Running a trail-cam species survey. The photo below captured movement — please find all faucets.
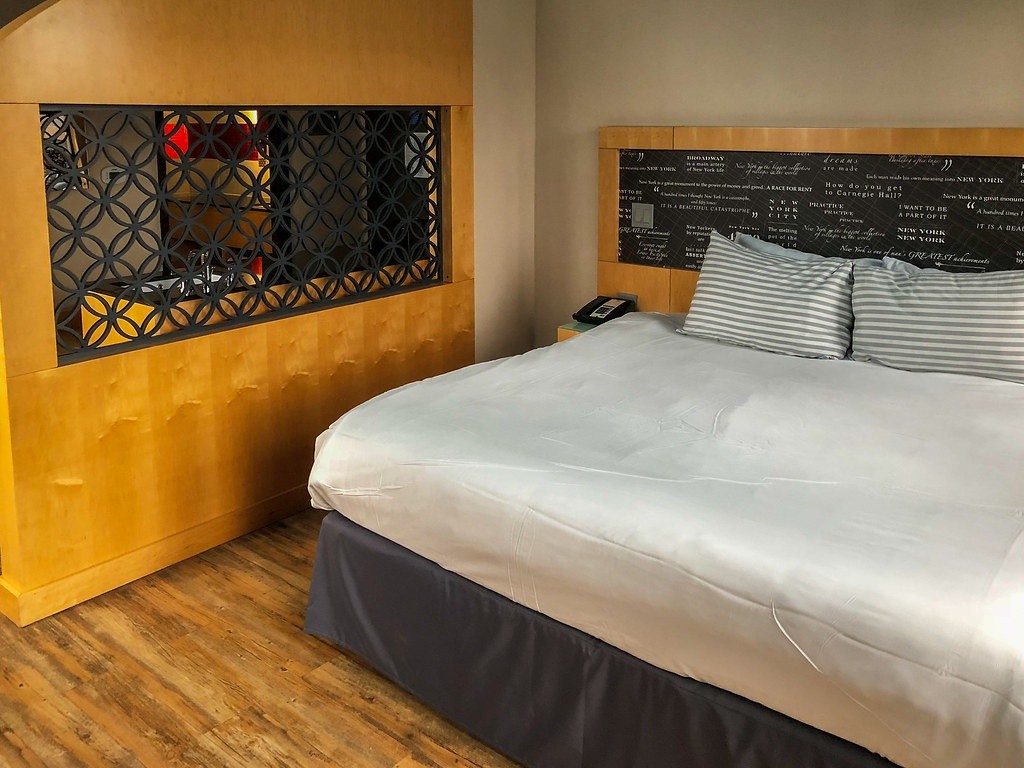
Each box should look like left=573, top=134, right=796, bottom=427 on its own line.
left=189, top=250, right=211, bottom=293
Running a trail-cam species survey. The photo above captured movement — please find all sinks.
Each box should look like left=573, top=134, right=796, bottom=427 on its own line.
left=110, top=269, right=244, bottom=292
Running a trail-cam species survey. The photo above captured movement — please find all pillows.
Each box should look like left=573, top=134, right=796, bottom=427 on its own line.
left=675, top=229, right=1024, bottom=385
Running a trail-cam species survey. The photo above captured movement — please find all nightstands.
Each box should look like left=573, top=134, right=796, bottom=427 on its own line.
left=557, top=321, right=597, bottom=342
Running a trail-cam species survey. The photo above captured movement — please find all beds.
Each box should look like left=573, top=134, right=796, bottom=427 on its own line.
left=304, top=310, right=1024, bottom=768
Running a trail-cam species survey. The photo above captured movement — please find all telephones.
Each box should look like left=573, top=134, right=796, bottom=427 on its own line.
left=572, top=295, right=633, bottom=326
